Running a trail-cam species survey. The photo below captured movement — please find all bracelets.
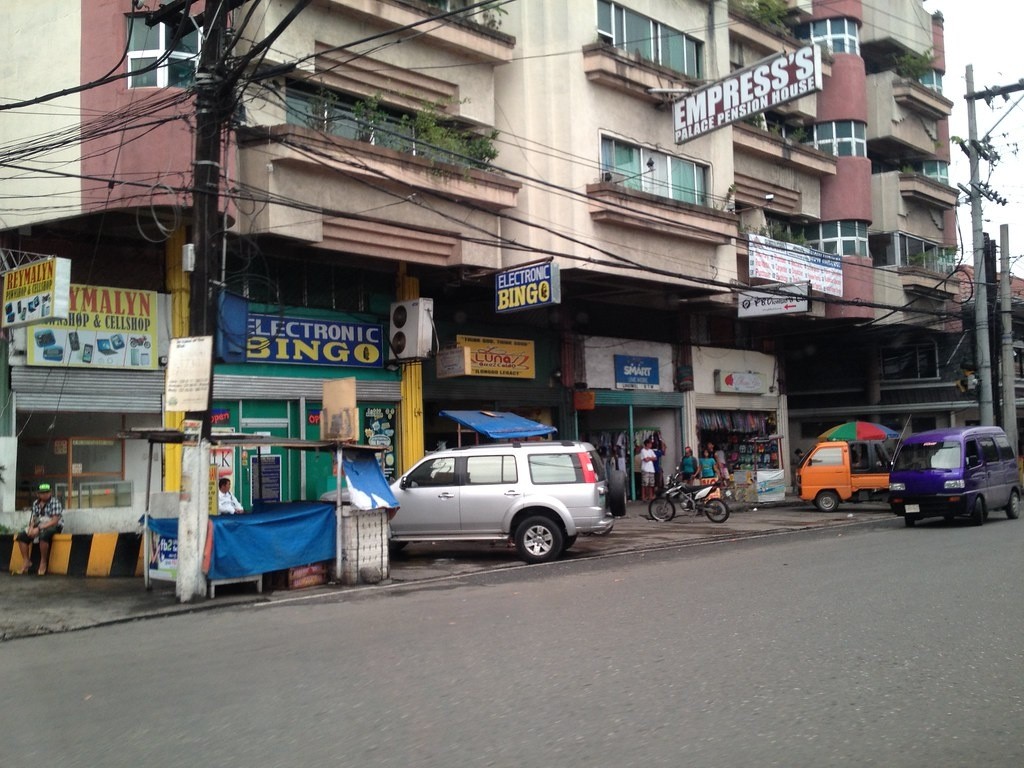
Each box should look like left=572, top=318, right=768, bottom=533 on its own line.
left=38, top=526, right=41, bottom=531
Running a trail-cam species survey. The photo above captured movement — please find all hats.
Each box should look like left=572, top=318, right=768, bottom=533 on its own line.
left=38, top=484, right=51, bottom=493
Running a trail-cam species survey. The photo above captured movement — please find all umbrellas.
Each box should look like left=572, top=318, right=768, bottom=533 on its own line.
left=817, top=420, right=900, bottom=442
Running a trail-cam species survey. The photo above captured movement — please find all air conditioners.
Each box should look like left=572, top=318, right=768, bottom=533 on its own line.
left=388, top=298, right=433, bottom=361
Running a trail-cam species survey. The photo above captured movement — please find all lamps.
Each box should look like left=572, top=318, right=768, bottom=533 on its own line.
left=735, top=193, right=774, bottom=213
left=613, top=157, right=654, bottom=183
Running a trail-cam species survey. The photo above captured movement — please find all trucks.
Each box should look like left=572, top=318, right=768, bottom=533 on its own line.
left=794, top=439, right=891, bottom=514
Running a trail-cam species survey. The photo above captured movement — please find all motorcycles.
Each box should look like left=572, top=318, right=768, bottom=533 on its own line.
left=648, top=465, right=730, bottom=524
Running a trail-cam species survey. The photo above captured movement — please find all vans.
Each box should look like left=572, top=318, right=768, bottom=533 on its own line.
left=886, top=424, right=1022, bottom=527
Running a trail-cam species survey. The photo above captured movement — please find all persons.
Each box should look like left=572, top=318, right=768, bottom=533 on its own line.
left=16, top=483, right=64, bottom=575
left=679, top=447, right=697, bottom=485
left=690, top=449, right=720, bottom=479
left=217, top=478, right=245, bottom=515
left=794, top=448, right=804, bottom=461
left=639, top=439, right=657, bottom=500
left=705, top=442, right=728, bottom=486
left=651, top=443, right=665, bottom=491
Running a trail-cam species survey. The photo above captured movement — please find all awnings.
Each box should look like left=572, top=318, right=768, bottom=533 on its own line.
left=439, top=409, right=557, bottom=439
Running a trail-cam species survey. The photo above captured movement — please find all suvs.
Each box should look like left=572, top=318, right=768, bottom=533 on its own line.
left=319, top=437, right=628, bottom=564
